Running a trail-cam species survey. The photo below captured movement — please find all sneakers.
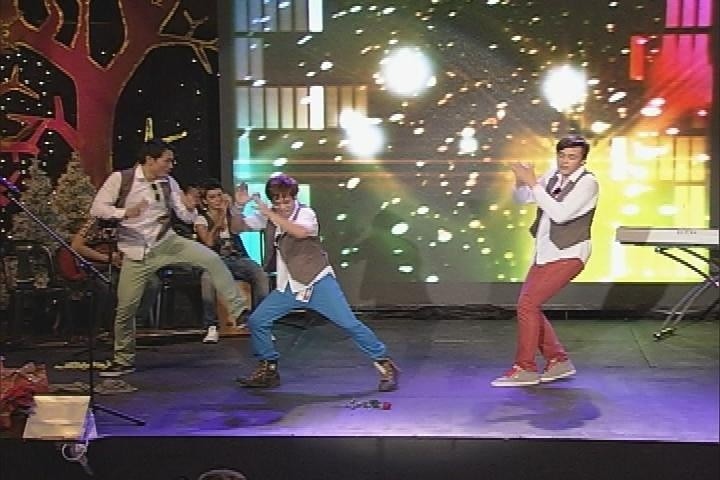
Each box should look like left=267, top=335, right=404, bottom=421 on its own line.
left=490, top=362, right=541, bottom=388
left=201, top=324, right=221, bottom=344
left=538, top=358, right=578, bottom=384
left=235, top=308, right=255, bottom=328
left=98, top=360, right=138, bottom=378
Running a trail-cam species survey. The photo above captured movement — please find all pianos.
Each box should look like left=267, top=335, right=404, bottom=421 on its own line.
left=615, top=226, right=720, bottom=245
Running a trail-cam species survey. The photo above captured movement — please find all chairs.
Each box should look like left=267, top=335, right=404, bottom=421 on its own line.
left=12, top=241, right=253, bottom=335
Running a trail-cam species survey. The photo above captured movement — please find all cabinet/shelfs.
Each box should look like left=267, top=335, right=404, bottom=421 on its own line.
left=616, top=244, right=720, bottom=339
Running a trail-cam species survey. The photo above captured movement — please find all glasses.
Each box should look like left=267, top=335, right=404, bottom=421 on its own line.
left=149, top=183, right=161, bottom=201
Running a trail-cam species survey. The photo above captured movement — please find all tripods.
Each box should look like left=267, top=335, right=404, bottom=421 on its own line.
left=0, top=185, right=148, bottom=426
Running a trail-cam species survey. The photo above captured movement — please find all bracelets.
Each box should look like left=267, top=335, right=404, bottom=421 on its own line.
left=230, top=201, right=245, bottom=217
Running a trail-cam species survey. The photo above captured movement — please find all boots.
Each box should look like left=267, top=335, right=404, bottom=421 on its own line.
left=238, top=359, right=282, bottom=390
left=376, top=354, right=399, bottom=392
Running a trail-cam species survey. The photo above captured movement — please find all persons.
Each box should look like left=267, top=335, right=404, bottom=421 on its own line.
left=230, top=176, right=402, bottom=394
left=194, top=182, right=278, bottom=345
left=490, top=135, right=600, bottom=388
left=88, top=137, right=258, bottom=379
left=67, top=208, right=125, bottom=351
